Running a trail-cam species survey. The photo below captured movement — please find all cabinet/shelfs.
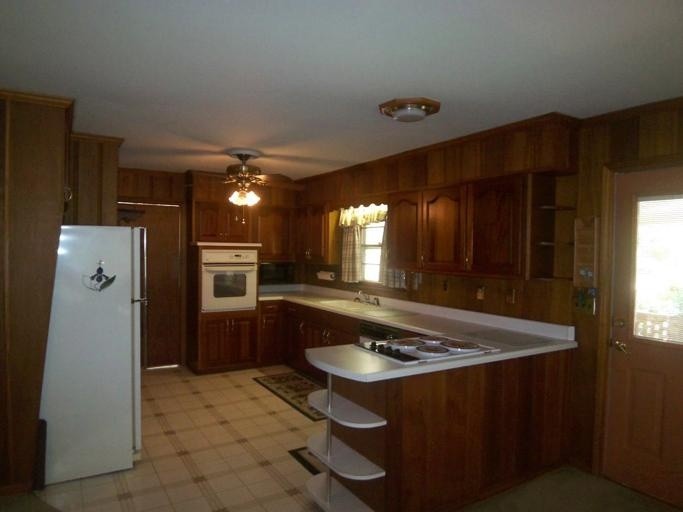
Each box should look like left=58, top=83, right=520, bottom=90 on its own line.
left=186, top=111, right=598, bottom=512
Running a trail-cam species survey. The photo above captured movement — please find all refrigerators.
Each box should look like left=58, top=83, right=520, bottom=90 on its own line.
left=37, top=224, right=150, bottom=489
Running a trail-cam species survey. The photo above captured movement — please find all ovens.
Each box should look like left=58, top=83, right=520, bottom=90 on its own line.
left=200, top=249, right=257, bottom=312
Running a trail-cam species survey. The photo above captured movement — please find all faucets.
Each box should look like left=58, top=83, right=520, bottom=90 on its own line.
left=362, top=292, right=370, bottom=303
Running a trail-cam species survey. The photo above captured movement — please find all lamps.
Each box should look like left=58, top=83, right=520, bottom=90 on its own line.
left=379, top=96, right=439, bottom=122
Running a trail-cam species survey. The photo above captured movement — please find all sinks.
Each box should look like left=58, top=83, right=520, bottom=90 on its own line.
left=319, top=299, right=380, bottom=311
